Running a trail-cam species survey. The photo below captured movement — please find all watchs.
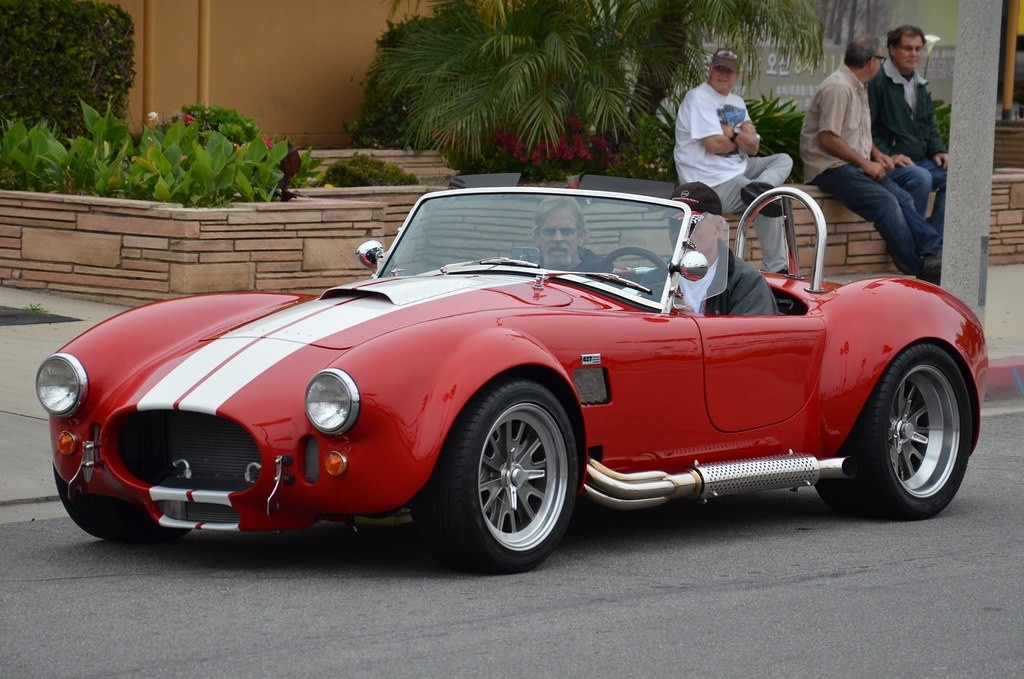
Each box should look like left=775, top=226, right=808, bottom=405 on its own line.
left=731, top=126, right=740, bottom=140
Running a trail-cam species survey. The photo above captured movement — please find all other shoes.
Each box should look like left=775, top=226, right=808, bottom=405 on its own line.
left=740, top=181, right=783, bottom=218
left=919, top=262, right=940, bottom=286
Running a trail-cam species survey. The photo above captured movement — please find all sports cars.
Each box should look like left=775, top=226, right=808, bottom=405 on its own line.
left=34, top=172, right=989, bottom=576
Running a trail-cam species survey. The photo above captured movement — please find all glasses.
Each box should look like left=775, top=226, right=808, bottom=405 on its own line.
left=538, top=225, right=581, bottom=236
left=867, top=54, right=887, bottom=65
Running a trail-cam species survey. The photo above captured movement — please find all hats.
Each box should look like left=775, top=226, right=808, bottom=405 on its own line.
left=660, top=181, right=722, bottom=220
left=713, top=48, right=738, bottom=72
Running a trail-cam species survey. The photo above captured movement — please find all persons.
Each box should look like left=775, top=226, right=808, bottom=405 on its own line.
left=528, top=196, right=615, bottom=274
left=673, top=48, right=793, bottom=274
left=639, top=181, right=779, bottom=317
left=867, top=25, right=949, bottom=235
left=799, top=37, right=943, bottom=286
